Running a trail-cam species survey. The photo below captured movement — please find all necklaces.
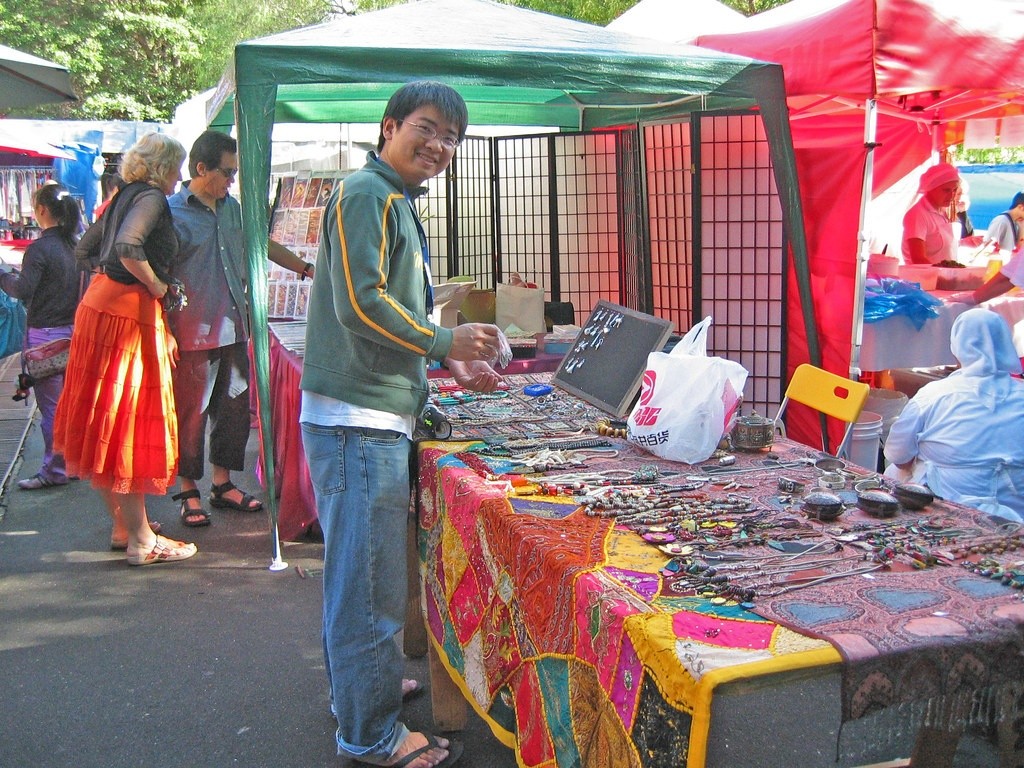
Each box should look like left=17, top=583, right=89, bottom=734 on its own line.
left=426, top=381, right=1024, bottom=611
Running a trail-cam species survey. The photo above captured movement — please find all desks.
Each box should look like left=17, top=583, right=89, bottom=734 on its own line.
left=859, top=296, right=1024, bottom=376
left=411, top=367, right=1024, bottom=768
left=249, top=322, right=575, bottom=542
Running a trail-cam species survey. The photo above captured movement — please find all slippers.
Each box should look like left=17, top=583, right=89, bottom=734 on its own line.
left=331, top=679, right=423, bottom=720
left=128, top=536, right=198, bottom=566
left=352, top=729, right=464, bottom=768
left=111, top=519, right=162, bottom=550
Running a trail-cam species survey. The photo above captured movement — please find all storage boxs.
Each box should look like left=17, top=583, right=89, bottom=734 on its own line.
left=543, top=332, right=577, bottom=355
left=510, top=337, right=537, bottom=358
left=553, top=323, right=581, bottom=334
left=505, top=330, right=538, bottom=338
left=908, top=264, right=990, bottom=291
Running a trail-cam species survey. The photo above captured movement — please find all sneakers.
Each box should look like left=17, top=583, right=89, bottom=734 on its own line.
left=19, top=471, right=54, bottom=489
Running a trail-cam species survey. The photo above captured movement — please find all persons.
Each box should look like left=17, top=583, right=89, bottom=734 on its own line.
left=988, top=192, right=1024, bottom=250
left=899, top=164, right=959, bottom=264
left=944, top=239, right=1024, bottom=305
left=54, top=133, right=197, bottom=566
left=883, top=308, right=1024, bottom=523
left=0, top=185, right=90, bottom=492
left=171, top=131, right=316, bottom=528
left=298, top=81, right=502, bottom=768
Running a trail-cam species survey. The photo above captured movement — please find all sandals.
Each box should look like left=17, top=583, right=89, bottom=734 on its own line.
left=172, top=488, right=210, bottom=527
left=209, top=479, right=262, bottom=511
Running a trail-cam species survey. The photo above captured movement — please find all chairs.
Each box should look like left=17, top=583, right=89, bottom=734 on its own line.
left=770, top=363, right=870, bottom=459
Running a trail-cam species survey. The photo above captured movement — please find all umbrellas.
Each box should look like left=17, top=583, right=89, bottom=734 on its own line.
left=1, top=46, right=78, bottom=116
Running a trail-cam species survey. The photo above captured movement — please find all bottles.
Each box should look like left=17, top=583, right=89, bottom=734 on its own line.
left=985, top=244, right=1003, bottom=282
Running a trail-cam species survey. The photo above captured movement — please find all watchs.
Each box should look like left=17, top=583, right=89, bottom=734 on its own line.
left=301, top=263, right=314, bottom=281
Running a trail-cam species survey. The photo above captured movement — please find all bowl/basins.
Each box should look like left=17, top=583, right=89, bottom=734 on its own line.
left=929, top=265, right=987, bottom=291
left=867, top=256, right=901, bottom=277
left=898, top=263, right=941, bottom=291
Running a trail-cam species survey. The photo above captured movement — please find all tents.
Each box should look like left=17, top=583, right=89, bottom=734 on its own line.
left=207, top=0, right=1024, bottom=570
left=0, top=119, right=171, bottom=271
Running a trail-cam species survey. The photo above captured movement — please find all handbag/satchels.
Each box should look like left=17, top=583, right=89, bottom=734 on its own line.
left=22, top=336, right=73, bottom=379
left=496, top=272, right=547, bottom=336
left=627, top=315, right=748, bottom=463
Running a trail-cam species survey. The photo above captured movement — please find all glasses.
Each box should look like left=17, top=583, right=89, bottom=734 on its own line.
left=398, top=119, right=459, bottom=150
left=217, top=165, right=238, bottom=178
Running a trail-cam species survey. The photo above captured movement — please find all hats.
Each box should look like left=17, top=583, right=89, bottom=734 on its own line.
left=918, top=162, right=959, bottom=193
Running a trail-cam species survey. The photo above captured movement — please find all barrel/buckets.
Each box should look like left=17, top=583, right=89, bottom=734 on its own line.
left=849, top=410, right=884, bottom=473
